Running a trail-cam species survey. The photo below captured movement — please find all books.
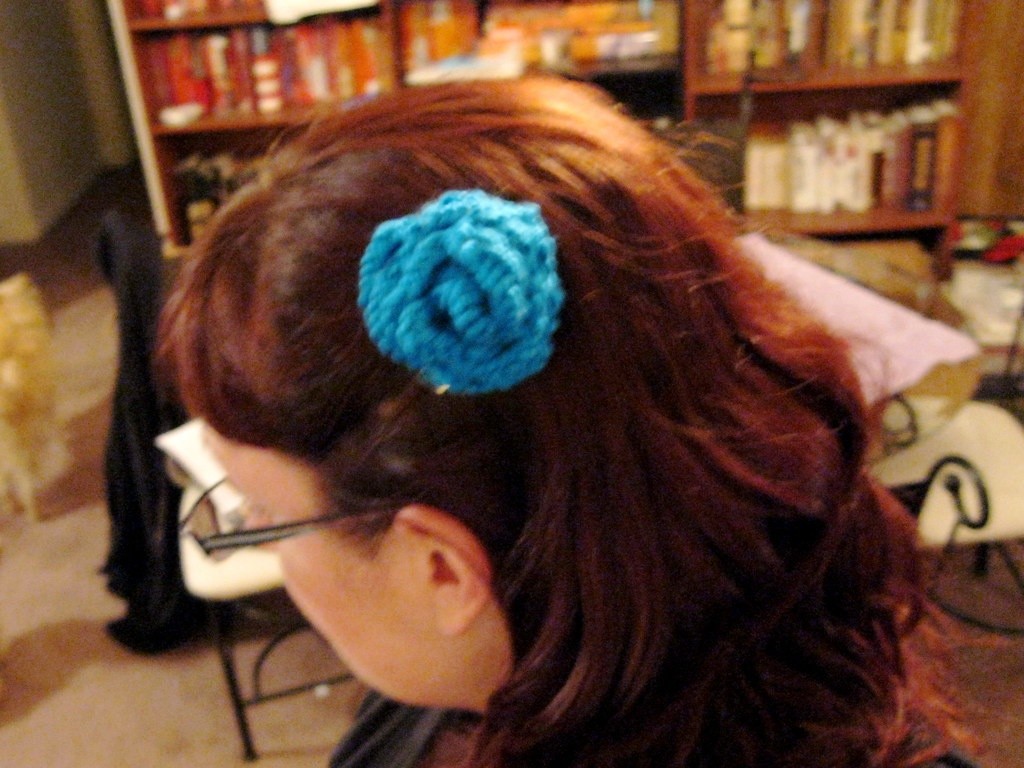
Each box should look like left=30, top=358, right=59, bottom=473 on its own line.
left=394, top=2, right=677, bottom=76
left=134, top=0, right=383, bottom=120
left=741, top=97, right=964, bottom=217
left=701, top=1, right=963, bottom=77
left=168, top=149, right=273, bottom=246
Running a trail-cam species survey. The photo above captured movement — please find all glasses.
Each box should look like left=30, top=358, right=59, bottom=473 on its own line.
left=177, top=478, right=394, bottom=562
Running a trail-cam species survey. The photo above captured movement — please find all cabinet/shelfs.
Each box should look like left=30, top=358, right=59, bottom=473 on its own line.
left=669, top=0, right=983, bottom=288
left=105, top=0, right=396, bottom=252
left=395, top=0, right=685, bottom=158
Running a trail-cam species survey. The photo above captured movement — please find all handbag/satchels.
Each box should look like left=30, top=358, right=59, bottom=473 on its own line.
left=732, top=228, right=979, bottom=459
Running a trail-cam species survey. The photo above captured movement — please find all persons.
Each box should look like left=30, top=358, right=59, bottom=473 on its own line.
left=158, top=77, right=982, bottom=768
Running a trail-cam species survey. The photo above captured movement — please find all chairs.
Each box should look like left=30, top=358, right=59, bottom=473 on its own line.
left=865, top=391, right=1024, bottom=645
left=177, top=486, right=357, bottom=764
